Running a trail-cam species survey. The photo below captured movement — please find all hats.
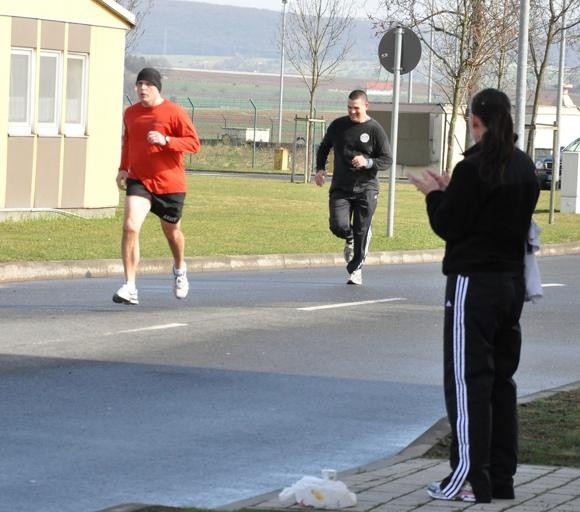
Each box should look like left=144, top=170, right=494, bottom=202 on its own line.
left=138, top=68, right=161, bottom=91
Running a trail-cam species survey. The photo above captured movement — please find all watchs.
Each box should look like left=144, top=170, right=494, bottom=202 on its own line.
left=165, top=135, right=170, bottom=144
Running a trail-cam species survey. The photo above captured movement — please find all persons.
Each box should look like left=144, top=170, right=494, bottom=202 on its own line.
left=409, top=89, right=540, bottom=505
left=112, top=67, right=201, bottom=304
left=315, top=90, right=393, bottom=285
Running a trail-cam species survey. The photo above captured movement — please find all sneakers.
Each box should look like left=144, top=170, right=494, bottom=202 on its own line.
left=344, top=240, right=355, bottom=263
left=344, top=269, right=362, bottom=285
left=173, top=261, right=189, bottom=299
left=427, top=481, right=476, bottom=502
left=113, top=284, right=139, bottom=305
